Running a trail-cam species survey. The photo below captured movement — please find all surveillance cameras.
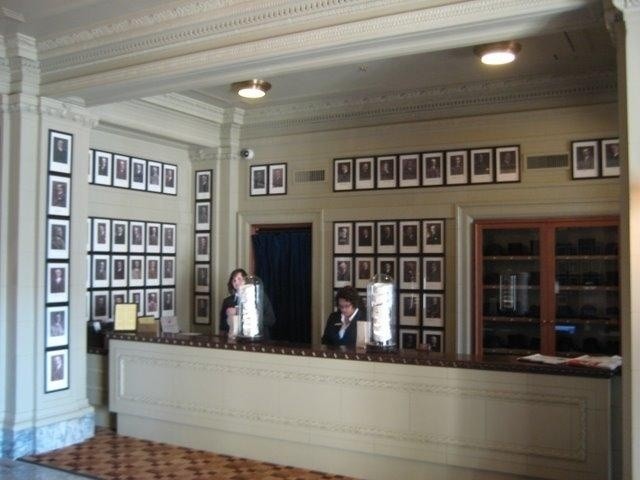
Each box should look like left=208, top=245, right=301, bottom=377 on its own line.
left=242, top=149, right=254, bottom=159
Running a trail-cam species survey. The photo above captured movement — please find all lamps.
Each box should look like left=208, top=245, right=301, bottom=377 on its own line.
left=231, top=79, right=271, bottom=99
left=473, top=41, right=522, bottom=65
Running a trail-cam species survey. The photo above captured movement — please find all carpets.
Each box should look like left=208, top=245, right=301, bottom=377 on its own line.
left=16, top=422, right=358, bottom=479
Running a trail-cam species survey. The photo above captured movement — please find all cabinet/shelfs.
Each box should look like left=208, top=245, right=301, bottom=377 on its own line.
left=471, top=215, right=623, bottom=359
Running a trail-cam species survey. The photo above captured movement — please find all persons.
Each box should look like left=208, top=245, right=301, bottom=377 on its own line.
left=51, top=134, right=68, bottom=381
left=272, top=168, right=282, bottom=188
left=404, top=158, right=417, bottom=178
left=95, top=155, right=175, bottom=318
left=451, top=155, right=463, bottom=175
left=219, top=268, right=276, bottom=342
left=197, top=174, right=210, bottom=319
left=427, top=157, right=441, bottom=178
left=381, top=159, right=392, bottom=180
left=475, top=152, right=490, bottom=175
left=501, top=151, right=515, bottom=173
left=336, top=224, right=442, bottom=350
left=254, top=170, right=264, bottom=188
left=320, top=285, right=369, bottom=347
left=606, top=142, right=621, bottom=168
left=360, top=162, right=370, bottom=179
left=576, top=146, right=594, bottom=169
left=338, top=162, right=351, bottom=182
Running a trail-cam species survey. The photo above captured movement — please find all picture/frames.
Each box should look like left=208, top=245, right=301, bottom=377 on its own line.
left=267, top=161, right=288, bottom=196
left=332, top=142, right=522, bottom=191
left=248, top=163, right=267, bottom=196
left=330, top=217, right=446, bottom=353
left=601, top=137, right=622, bottom=178
left=87, top=148, right=179, bottom=196
left=44, top=128, right=74, bottom=394
left=87, top=215, right=178, bottom=325
left=192, top=168, right=213, bottom=325
left=570, top=138, right=601, bottom=179
